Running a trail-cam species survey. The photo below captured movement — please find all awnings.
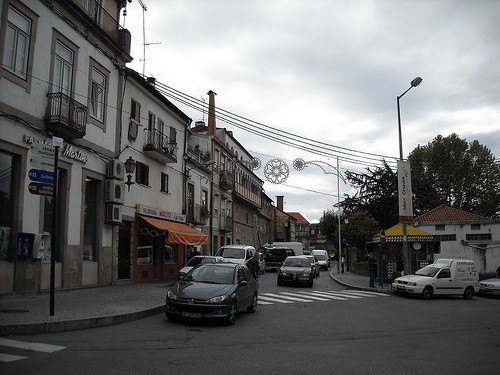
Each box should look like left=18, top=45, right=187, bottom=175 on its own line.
left=141, top=216, right=208, bottom=245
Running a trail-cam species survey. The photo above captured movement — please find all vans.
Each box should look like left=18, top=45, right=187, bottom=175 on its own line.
left=213, top=244, right=260, bottom=280
left=311, top=250, right=331, bottom=269
left=256, top=241, right=303, bottom=272
left=136, top=245, right=174, bottom=264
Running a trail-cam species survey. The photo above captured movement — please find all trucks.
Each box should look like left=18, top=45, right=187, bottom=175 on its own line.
left=392, top=258, right=480, bottom=299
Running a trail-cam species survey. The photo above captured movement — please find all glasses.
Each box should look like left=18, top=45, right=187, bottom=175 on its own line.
left=371, top=254, right=374, bottom=255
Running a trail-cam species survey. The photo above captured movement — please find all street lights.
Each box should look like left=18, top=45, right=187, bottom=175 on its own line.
left=396, top=76, right=423, bottom=275
left=184, top=159, right=216, bottom=267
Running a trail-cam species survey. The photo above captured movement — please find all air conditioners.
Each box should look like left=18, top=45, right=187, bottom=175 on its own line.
left=107, top=161, right=124, bottom=181
left=221, top=173, right=232, bottom=185
left=106, top=179, right=125, bottom=204
left=107, top=204, right=122, bottom=223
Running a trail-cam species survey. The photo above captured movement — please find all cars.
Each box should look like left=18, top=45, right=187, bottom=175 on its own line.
left=165, top=255, right=259, bottom=326
left=277, top=254, right=320, bottom=287
left=257, top=252, right=266, bottom=274
left=478, top=277, right=500, bottom=296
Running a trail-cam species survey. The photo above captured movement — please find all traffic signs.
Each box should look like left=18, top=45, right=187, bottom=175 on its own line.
left=28, top=182, right=54, bottom=195
left=27, top=168, right=54, bottom=183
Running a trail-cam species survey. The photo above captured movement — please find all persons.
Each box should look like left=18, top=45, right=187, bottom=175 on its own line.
left=186, top=244, right=194, bottom=265
left=396, top=256, right=404, bottom=272
left=246, top=252, right=260, bottom=281
left=367, top=253, right=377, bottom=288
left=335, top=249, right=346, bottom=273
left=191, top=246, right=202, bottom=264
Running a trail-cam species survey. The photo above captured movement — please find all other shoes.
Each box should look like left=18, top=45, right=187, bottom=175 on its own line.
left=370, top=286, right=375, bottom=288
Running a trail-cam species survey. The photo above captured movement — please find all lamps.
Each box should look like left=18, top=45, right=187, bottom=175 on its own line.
left=186, top=160, right=216, bottom=172
left=123, top=156, right=136, bottom=192
